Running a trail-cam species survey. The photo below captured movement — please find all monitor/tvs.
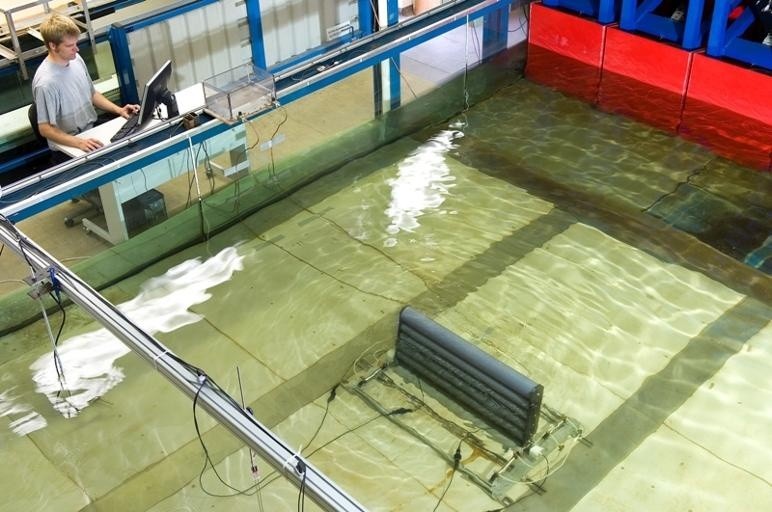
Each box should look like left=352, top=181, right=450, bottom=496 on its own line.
left=137, top=59, right=181, bottom=126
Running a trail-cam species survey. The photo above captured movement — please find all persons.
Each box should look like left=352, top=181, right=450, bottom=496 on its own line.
left=31, top=15, right=141, bottom=153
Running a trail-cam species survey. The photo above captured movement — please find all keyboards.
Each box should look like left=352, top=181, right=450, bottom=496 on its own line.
left=111, top=110, right=140, bottom=143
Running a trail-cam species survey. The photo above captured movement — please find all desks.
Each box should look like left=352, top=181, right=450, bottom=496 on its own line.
left=55, top=81, right=250, bottom=246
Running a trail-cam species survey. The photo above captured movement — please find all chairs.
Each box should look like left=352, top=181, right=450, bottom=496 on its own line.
left=28, top=102, right=104, bottom=228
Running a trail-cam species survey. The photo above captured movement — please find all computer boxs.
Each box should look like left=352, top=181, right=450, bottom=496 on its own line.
left=121, top=189, right=168, bottom=239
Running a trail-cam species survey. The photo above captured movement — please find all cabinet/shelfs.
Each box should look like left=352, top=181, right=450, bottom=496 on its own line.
left=0, top=0, right=98, bottom=81
